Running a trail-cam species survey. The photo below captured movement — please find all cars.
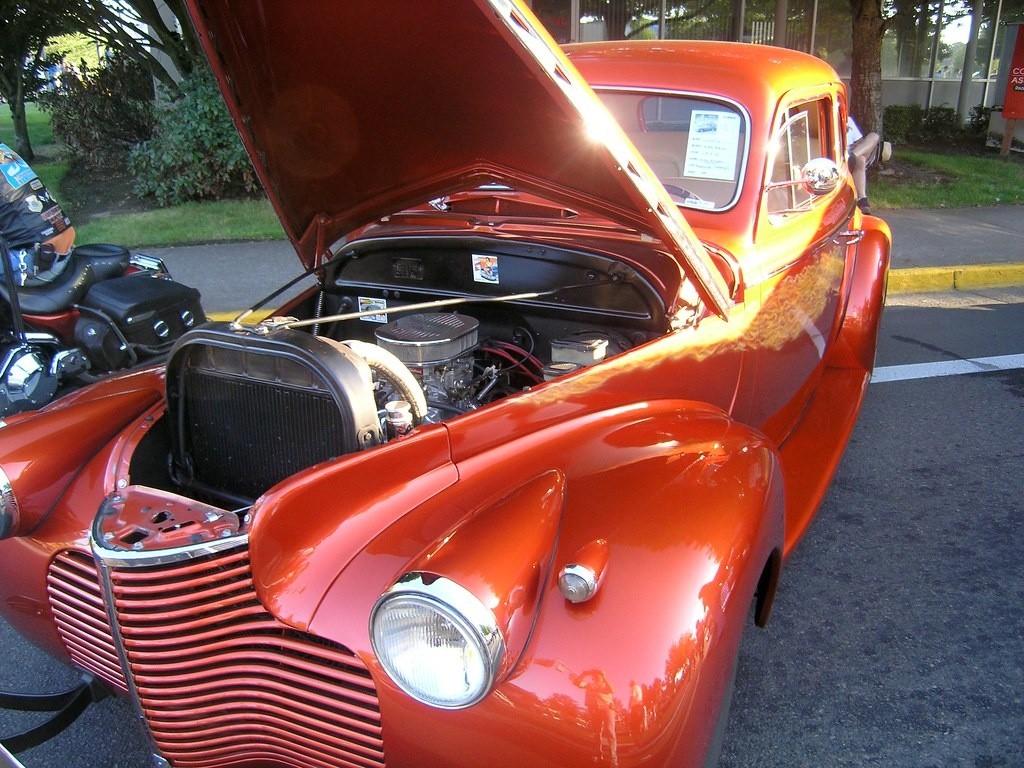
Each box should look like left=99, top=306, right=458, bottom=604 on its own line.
left=0, top=0, right=891, bottom=767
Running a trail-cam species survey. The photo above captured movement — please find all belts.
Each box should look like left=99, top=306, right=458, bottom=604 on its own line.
left=52, top=252, right=69, bottom=262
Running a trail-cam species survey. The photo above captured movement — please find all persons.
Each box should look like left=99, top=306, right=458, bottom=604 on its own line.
left=845, top=116, right=880, bottom=214
left=0, top=142, right=75, bottom=342
left=953, top=68, right=961, bottom=78
left=972, top=68, right=983, bottom=78
left=992, top=70, right=997, bottom=74
left=936, top=66, right=950, bottom=78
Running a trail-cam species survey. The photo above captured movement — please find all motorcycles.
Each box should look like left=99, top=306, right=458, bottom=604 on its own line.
left=0, top=241, right=208, bottom=421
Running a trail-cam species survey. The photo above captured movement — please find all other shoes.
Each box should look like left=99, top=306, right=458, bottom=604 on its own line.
left=858, top=198, right=872, bottom=214
left=848, top=153, right=857, bottom=173
